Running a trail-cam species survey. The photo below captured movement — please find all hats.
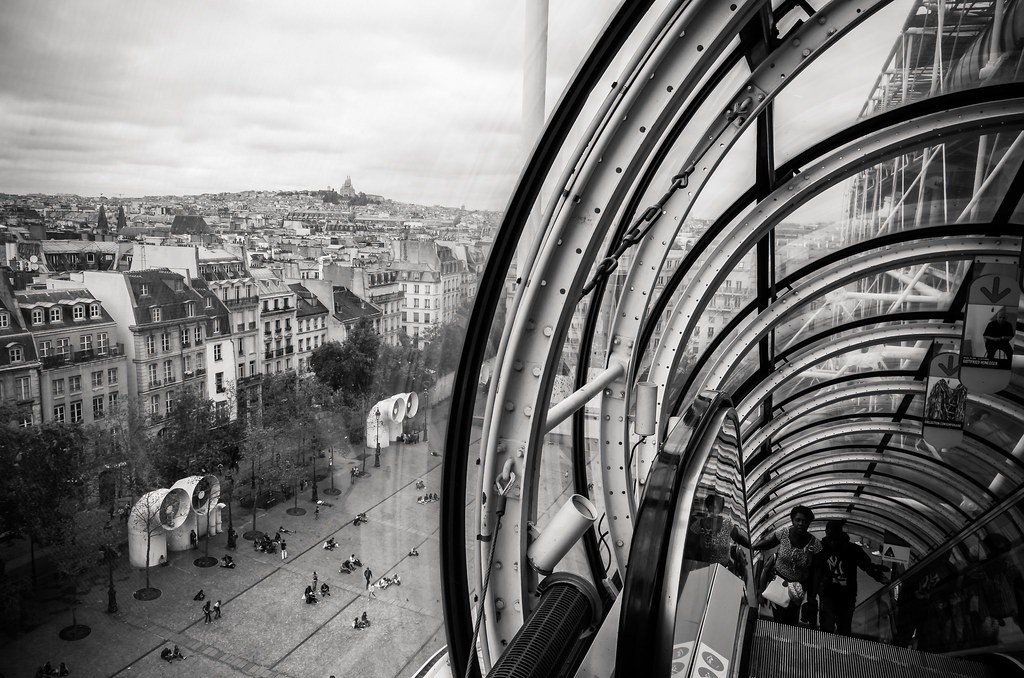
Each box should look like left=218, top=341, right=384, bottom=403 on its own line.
left=788, top=582, right=804, bottom=602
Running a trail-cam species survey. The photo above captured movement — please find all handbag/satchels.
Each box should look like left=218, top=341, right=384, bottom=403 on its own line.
left=302, top=594, right=307, bottom=599
left=982, top=573, right=1018, bottom=619
left=761, top=575, right=790, bottom=608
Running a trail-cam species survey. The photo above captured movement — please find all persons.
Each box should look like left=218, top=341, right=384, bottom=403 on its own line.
left=300, top=478, right=308, bottom=492
left=323, top=537, right=340, bottom=551
left=688, top=493, right=751, bottom=581
left=752, top=504, right=891, bottom=637
left=350, top=466, right=359, bottom=484
left=305, top=571, right=330, bottom=604
left=315, top=496, right=325, bottom=505
left=353, top=512, right=368, bottom=527
left=160, top=644, right=188, bottom=664
left=982, top=311, right=1014, bottom=360
left=193, top=589, right=223, bottom=624
left=315, top=506, right=320, bottom=518
left=220, top=529, right=237, bottom=568
left=351, top=612, right=370, bottom=629
left=254, top=526, right=296, bottom=559
left=367, top=573, right=401, bottom=602
left=400, top=432, right=418, bottom=444
left=159, top=554, right=170, bottom=567
left=34, top=660, right=71, bottom=678
left=339, top=554, right=364, bottom=574
left=364, top=567, right=372, bottom=589
left=190, top=530, right=198, bottom=549
left=416, top=480, right=440, bottom=504
left=890, top=530, right=1022, bottom=664
left=410, top=547, right=417, bottom=556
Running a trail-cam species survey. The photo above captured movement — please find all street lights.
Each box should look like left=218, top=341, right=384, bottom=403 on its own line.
left=423, top=387, right=430, bottom=443
left=374, top=407, right=382, bottom=468
left=311, top=435, right=320, bottom=502
left=226, top=469, right=238, bottom=548
left=104, top=520, right=119, bottom=614
